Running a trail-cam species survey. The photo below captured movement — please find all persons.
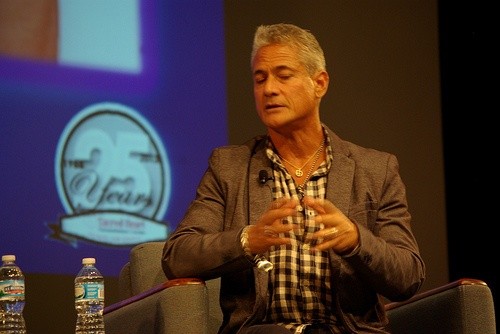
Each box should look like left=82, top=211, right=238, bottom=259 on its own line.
left=162, top=23, right=426, bottom=334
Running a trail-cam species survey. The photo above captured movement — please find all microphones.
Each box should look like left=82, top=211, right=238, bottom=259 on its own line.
left=258, top=169, right=274, bottom=183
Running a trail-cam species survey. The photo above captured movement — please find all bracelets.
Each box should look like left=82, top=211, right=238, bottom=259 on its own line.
left=240, top=224, right=272, bottom=272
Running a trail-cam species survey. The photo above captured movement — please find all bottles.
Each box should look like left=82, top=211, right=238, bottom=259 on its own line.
left=0, top=255, right=28, bottom=334
left=74, top=258, right=105, bottom=334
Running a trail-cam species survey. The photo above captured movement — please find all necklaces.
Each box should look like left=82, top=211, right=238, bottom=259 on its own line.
left=276, top=132, right=326, bottom=199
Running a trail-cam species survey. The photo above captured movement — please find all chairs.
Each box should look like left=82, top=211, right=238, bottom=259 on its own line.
left=103, top=241, right=497, bottom=334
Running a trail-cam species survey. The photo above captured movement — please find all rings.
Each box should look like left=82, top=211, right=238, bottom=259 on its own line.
left=331, top=225, right=337, bottom=238
